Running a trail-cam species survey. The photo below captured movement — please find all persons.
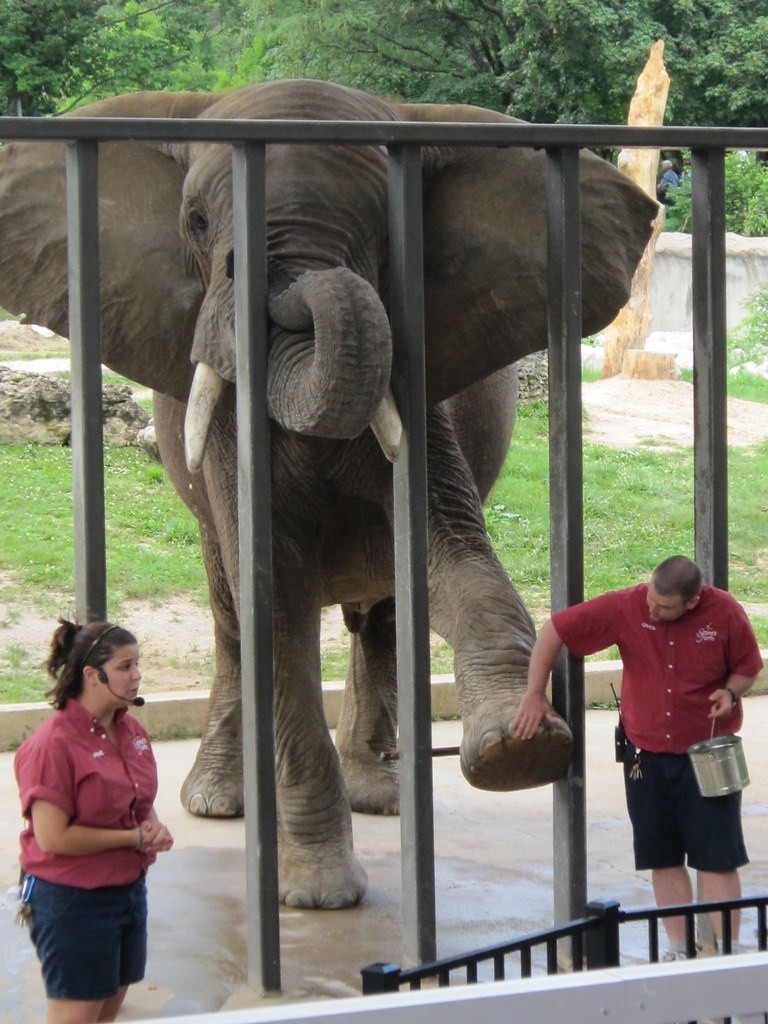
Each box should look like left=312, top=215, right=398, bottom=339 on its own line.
left=657, top=160, right=680, bottom=206
left=511, top=555, right=764, bottom=963
left=13, top=616, right=174, bottom=1024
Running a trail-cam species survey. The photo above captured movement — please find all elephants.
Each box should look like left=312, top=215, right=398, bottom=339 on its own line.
left=0, top=77, right=660, bottom=913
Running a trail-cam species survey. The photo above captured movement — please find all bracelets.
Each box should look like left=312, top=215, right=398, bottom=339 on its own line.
left=723, top=686, right=736, bottom=706
left=135, top=828, right=143, bottom=850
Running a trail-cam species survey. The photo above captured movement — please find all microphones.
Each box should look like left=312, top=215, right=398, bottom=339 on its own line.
left=97, top=666, right=145, bottom=706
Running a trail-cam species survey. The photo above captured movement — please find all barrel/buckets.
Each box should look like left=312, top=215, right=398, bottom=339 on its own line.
left=687, top=736, right=750, bottom=797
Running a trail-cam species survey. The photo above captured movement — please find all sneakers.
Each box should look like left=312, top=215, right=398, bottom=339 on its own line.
left=660, top=947, right=701, bottom=962
